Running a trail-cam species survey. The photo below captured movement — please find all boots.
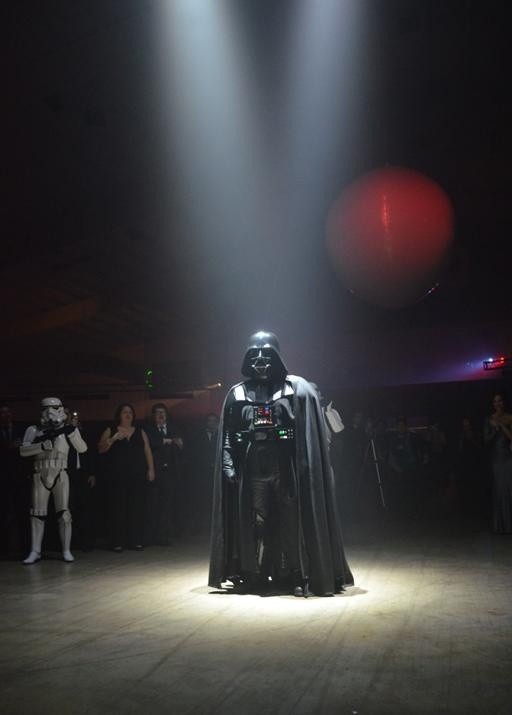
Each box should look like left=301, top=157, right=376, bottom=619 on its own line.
left=22, top=517, right=44, bottom=563
left=55, top=510, right=74, bottom=562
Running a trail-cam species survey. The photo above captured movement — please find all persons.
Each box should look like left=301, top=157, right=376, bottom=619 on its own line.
left=20, top=397, right=88, bottom=564
left=2, top=404, right=220, bottom=561
left=485, top=392, right=512, bottom=535
left=331, top=410, right=484, bottom=529
left=208, top=331, right=355, bottom=597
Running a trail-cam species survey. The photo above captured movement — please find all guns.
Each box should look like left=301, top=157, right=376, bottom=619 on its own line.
left=31, top=425, right=75, bottom=445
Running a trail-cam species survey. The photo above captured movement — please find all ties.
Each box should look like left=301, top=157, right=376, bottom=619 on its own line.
left=160, top=426, right=165, bottom=435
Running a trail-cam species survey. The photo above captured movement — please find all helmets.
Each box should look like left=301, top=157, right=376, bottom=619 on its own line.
left=240, top=331, right=288, bottom=380
left=38, top=397, right=68, bottom=424
